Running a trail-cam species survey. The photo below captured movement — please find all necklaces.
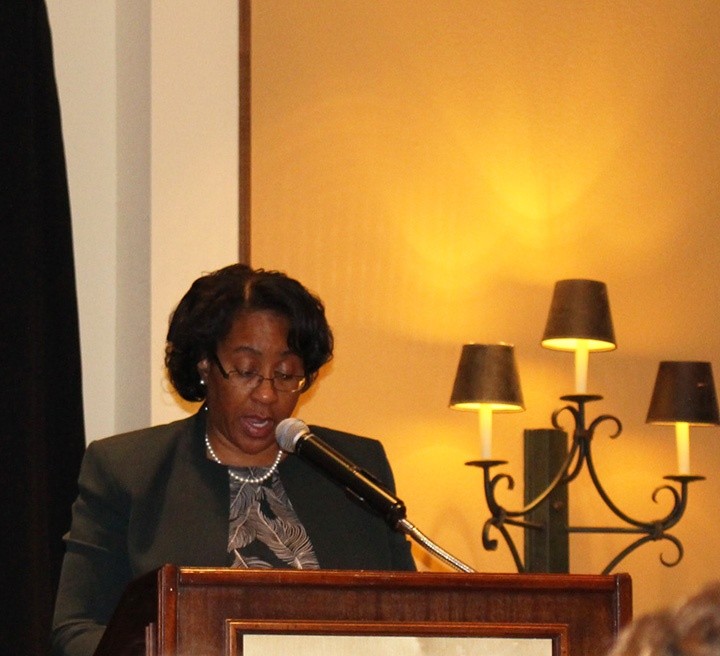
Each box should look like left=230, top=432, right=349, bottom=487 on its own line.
left=205, top=434, right=283, bottom=483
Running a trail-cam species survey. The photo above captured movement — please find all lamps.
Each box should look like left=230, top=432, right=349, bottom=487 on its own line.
left=448, top=279, right=720, bottom=576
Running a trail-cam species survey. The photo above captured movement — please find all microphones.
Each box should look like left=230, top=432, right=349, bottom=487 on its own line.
left=275, top=418, right=406, bottom=522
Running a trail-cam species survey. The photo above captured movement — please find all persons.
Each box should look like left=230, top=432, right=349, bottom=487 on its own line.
left=51, top=264, right=417, bottom=656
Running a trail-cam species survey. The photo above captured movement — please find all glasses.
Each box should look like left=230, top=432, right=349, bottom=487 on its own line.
left=208, top=345, right=307, bottom=392
